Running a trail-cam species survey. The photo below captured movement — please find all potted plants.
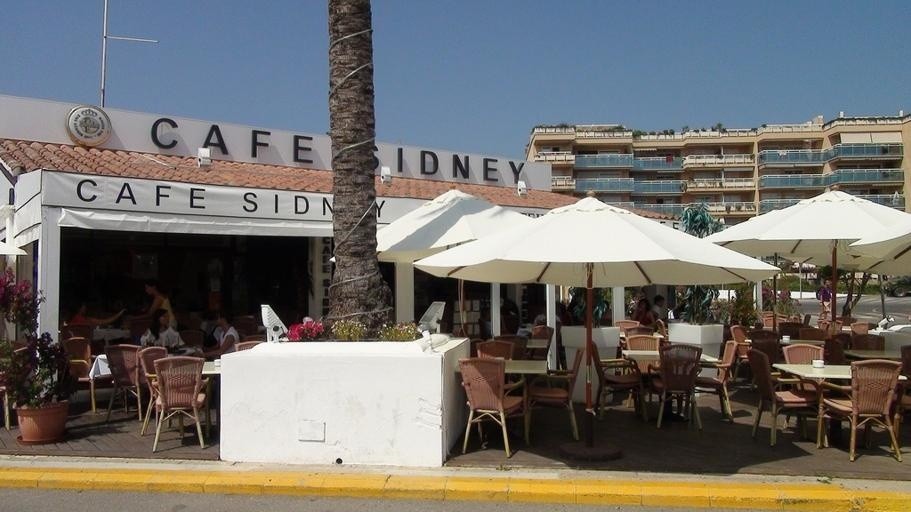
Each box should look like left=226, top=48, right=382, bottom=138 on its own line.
left=7, top=332, right=77, bottom=444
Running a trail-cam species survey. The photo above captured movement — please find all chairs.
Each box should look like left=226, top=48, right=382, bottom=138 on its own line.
left=782, top=343, right=844, bottom=433
left=817, top=358, right=904, bottom=461
left=588, top=318, right=739, bottom=431
left=861, top=343, right=911, bottom=451
left=474, top=324, right=554, bottom=363
left=746, top=349, right=830, bottom=449
left=102, top=343, right=206, bottom=452
left=729, top=316, right=911, bottom=387
left=0, top=332, right=96, bottom=431
left=456, top=358, right=524, bottom=458
left=524, top=347, right=586, bottom=444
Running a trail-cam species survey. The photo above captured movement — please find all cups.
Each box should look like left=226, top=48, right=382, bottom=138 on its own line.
left=214, top=358, right=221, bottom=367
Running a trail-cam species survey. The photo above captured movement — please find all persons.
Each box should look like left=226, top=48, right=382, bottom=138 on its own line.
left=67, top=281, right=240, bottom=360
left=500, top=295, right=667, bottom=337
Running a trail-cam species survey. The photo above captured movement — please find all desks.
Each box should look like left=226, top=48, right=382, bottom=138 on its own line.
left=455, top=357, right=548, bottom=378
left=771, top=361, right=909, bottom=381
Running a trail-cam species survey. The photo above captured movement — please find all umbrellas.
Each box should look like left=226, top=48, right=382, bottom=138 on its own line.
left=412, top=188, right=781, bottom=447
left=699, top=186, right=911, bottom=328
left=329, top=184, right=535, bottom=337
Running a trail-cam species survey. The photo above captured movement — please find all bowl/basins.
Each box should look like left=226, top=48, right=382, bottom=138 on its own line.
left=783, top=335, right=791, bottom=341
left=813, top=359, right=825, bottom=367
